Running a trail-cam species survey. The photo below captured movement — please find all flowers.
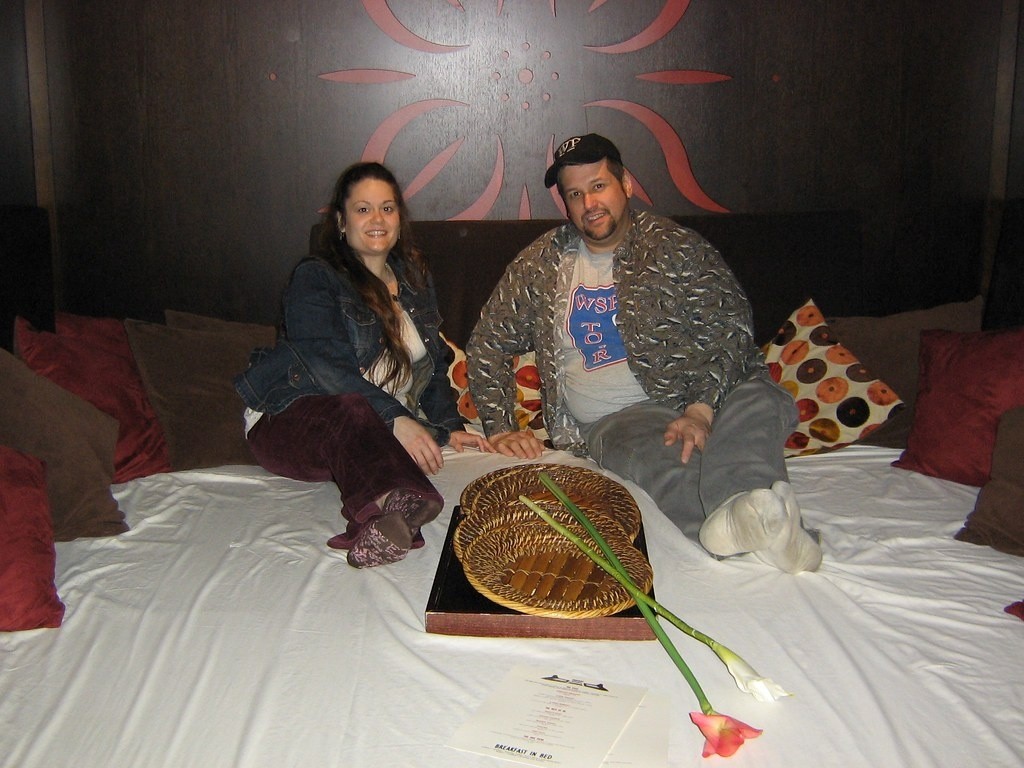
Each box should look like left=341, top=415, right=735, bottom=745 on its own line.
left=516, top=470, right=793, bottom=759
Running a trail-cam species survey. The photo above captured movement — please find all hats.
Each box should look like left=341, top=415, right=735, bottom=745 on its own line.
left=544, top=134, right=623, bottom=188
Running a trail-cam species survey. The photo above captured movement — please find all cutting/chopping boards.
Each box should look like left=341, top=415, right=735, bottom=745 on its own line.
left=425, top=503, right=658, bottom=641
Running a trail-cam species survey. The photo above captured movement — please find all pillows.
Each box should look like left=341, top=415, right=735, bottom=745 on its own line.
left=0, top=346, right=129, bottom=545
left=825, top=302, right=982, bottom=449
left=0, top=442, right=66, bottom=634
left=438, top=331, right=549, bottom=437
left=891, top=329, right=1024, bottom=488
left=761, top=296, right=907, bottom=459
left=953, top=406, right=1024, bottom=557
left=11, top=309, right=278, bottom=472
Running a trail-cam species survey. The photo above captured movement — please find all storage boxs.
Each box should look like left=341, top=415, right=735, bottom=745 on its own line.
left=425, top=500, right=658, bottom=642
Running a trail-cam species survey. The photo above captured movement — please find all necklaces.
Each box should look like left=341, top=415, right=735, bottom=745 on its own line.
left=384, top=263, right=398, bottom=301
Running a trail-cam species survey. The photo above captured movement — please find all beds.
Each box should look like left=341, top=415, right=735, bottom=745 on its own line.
left=0, top=208, right=1024, bottom=768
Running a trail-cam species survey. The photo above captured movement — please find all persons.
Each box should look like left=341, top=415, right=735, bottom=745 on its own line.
left=466, top=133, right=824, bottom=573
left=234, top=162, right=495, bottom=568
